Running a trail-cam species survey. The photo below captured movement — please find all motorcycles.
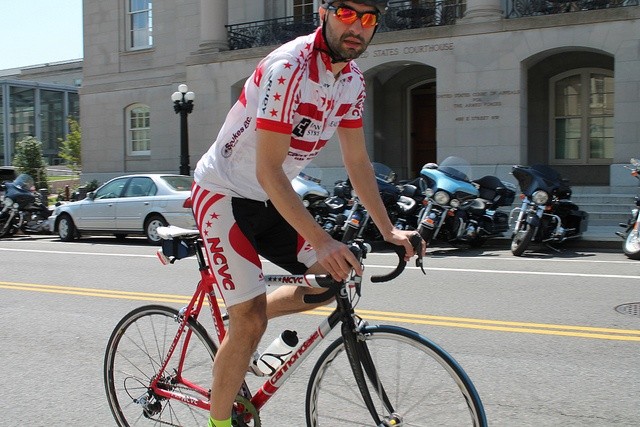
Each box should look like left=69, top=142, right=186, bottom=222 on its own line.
left=0, top=172, right=40, bottom=238
left=615, top=158, right=640, bottom=259
left=290, top=176, right=329, bottom=230
left=341, top=161, right=435, bottom=254
left=508, top=165, right=588, bottom=256
left=415, top=157, right=515, bottom=249
left=311, top=177, right=353, bottom=244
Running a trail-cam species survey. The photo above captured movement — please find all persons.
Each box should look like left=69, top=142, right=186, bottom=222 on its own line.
left=189, top=0, right=429, bottom=426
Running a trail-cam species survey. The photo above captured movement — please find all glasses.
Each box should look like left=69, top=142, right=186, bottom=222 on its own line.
left=321, top=4, right=378, bottom=29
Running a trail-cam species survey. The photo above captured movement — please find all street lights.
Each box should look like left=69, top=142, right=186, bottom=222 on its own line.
left=171, top=83, right=196, bottom=177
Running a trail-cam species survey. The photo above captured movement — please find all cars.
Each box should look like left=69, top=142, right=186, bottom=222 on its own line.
left=47, top=174, right=196, bottom=245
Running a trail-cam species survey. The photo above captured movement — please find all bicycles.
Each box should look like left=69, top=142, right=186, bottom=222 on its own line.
left=103, top=228, right=486, bottom=427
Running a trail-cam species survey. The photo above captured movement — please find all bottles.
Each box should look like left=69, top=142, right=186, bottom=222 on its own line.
left=256, top=330, right=299, bottom=375
